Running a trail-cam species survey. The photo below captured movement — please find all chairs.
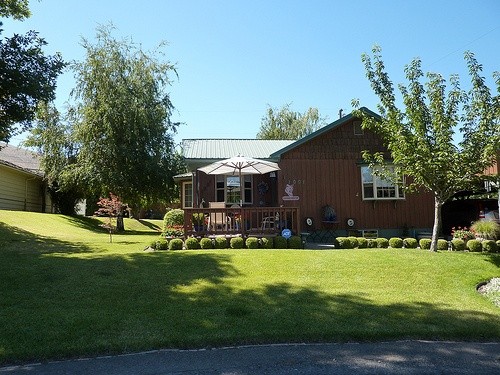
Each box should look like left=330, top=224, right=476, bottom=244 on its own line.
left=305, top=217, right=323, bottom=243
left=343, top=218, right=358, bottom=238
left=261, top=204, right=284, bottom=230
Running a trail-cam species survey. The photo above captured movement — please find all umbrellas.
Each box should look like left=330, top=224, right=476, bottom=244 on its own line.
left=197, top=153, right=281, bottom=207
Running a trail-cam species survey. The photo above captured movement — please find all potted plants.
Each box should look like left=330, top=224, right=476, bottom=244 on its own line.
left=323, top=206, right=337, bottom=221
left=163, top=208, right=205, bottom=232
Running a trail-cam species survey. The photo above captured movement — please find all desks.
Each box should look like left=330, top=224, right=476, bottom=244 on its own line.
left=320, top=221, right=341, bottom=242
